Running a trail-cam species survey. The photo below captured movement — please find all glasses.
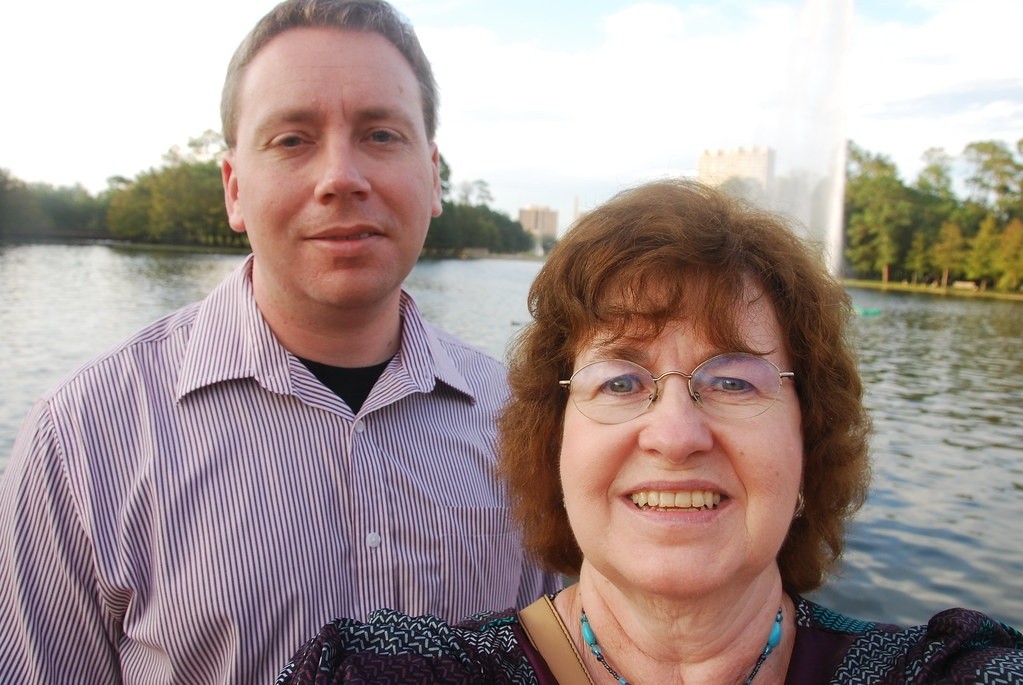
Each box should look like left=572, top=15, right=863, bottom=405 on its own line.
left=556, top=351, right=803, bottom=426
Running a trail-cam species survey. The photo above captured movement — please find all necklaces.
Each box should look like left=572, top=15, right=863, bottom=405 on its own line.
left=580, top=607, right=782, bottom=684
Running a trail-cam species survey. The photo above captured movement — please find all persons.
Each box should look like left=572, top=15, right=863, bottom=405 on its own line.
left=0, top=0, right=565, bottom=685
left=275, top=179, right=1023, bottom=684
